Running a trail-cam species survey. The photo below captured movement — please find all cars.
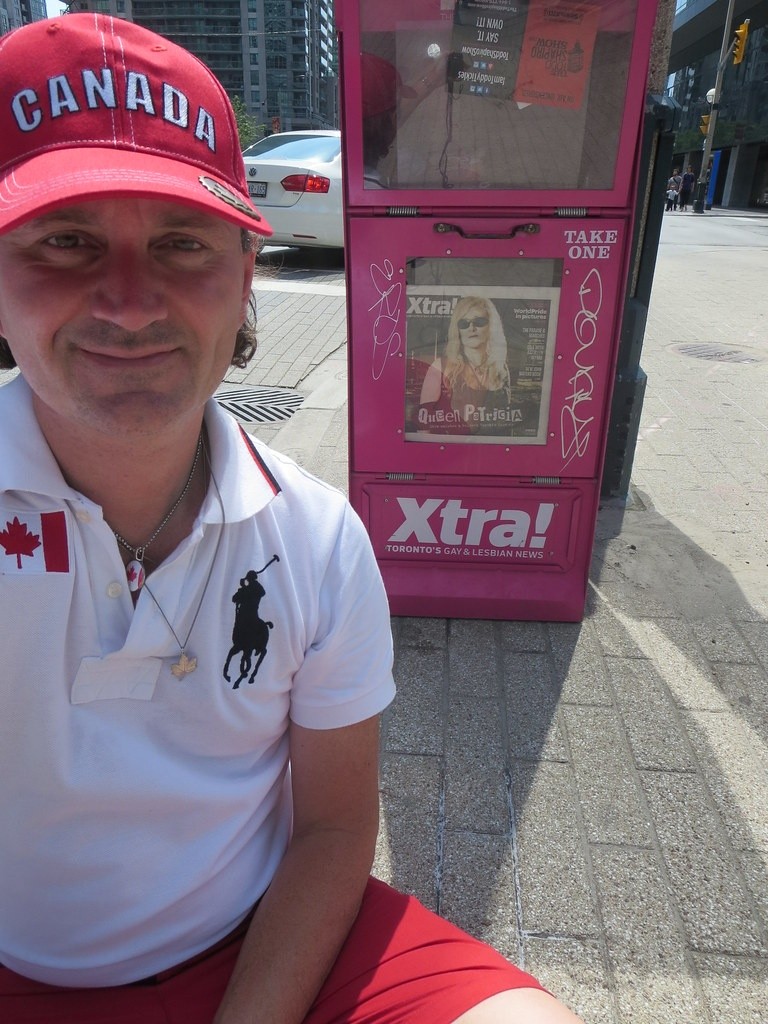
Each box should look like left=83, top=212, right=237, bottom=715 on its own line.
left=241, top=130, right=346, bottom=254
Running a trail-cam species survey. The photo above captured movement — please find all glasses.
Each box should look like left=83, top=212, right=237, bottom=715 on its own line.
left=457, top=318, right=488, bottom=328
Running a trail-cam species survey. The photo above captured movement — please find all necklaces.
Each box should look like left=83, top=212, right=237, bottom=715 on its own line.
left=139, top=448, right=226, bottom=682
left=467, top=357, right=489, bottom=391
left=112, top=429, right=204, bottom=593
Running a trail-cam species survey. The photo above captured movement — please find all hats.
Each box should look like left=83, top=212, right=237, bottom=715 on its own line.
left=0, top=13, right=274, bottom=240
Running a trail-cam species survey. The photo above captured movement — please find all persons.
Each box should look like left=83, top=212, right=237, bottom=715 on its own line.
left=358, top=50, right=419, bottom=189
left=679, top=165, right=695, bottom=212
left=668, top=169, right=682, bottom=211
left=0, top=13, right=593, bottom=1024
left=665, top=185, right=680, bottom=211
left=414, top=296, right=532, bottom=435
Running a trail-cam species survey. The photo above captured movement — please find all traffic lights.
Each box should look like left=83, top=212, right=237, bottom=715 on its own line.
left=732, top=23, right=748, bottom=64
left=700, top=115, right=710, bottom=135
